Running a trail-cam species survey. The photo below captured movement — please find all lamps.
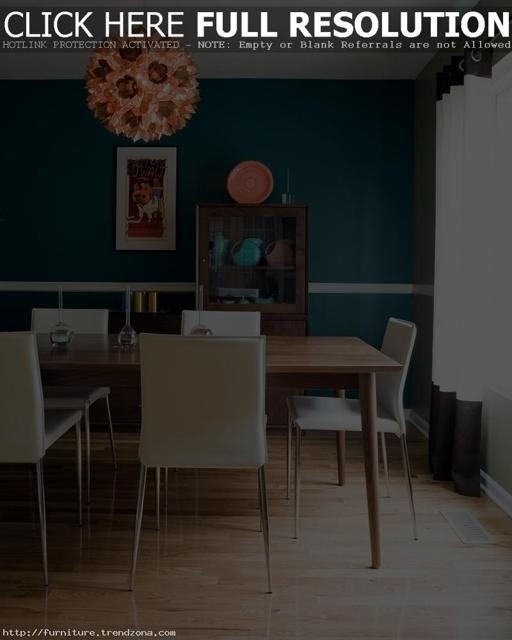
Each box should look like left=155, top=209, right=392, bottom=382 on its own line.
left=82, top=27, right=199, bottom=143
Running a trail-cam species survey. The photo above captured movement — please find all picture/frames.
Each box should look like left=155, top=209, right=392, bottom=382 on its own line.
left=114, top=147, right=178, bottom=252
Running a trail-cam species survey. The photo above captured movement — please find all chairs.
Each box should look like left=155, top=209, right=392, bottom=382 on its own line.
left=0, top=308, right=275, bottom=595
left=286, top=317, right=420, bottom=542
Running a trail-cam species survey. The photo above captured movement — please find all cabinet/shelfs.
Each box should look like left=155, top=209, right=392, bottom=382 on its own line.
left=198, top=205, right=305, bottom=314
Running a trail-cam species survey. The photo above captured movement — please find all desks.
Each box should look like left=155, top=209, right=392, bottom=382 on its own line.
left=37, top=335, right=401, bottom=571
